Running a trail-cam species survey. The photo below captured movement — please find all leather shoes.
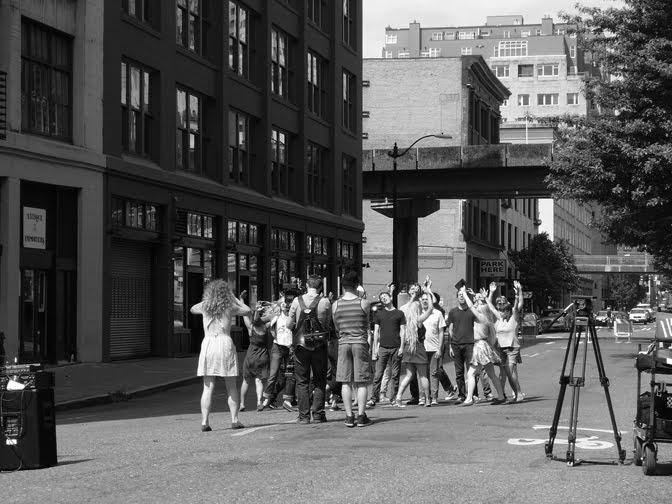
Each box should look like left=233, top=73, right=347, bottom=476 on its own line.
left=232, top=422, right=245, bottom=429
left=201, top=425, right=212, bottom=432
left=269, top=404, right=277, bottom=409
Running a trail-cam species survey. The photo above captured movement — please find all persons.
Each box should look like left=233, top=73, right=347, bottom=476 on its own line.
left=190, top=277, right=252, bottom=432
left=286, top=272, right=379, bottom=428
left=367, top=275, right=525, bottom=407
left=240, top=289, right=314, bottom=412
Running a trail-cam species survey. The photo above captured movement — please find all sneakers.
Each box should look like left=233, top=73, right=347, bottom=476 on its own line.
left=239, top=404, right=246, bottom=411
left=283, top=403, right=295, bottom=412
left=295, top=417, right=310, bottom=425
left=325, top=385, right=526, bottom=413
left=357, top=415, right=374, bottom=428
left=258, top=403, right=266, bottom=411
left=344, top=414, right=355, bottom=427
left=313, top=418, right=327, bottom=423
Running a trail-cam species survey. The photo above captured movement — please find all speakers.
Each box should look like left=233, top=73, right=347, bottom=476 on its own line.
left=2, top=388, right=57, bottom=470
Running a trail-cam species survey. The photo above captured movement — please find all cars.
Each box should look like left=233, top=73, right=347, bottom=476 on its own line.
left=594, top=300, right=657, bottom=325
left=539, top=309, right=571, bottom=332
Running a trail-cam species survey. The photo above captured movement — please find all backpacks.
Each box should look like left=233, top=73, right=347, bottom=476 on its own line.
left=292, top=294, right=330, bottom=365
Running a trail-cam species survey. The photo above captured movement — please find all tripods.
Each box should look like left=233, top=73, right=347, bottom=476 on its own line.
left=544, top=317, right=627, bottom=465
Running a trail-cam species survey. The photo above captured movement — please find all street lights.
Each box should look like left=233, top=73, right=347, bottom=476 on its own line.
left=386, top=131, right=453, bottom=311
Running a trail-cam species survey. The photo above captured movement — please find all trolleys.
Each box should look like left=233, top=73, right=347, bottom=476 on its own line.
left=631, top=334, right=672, bottom=476
